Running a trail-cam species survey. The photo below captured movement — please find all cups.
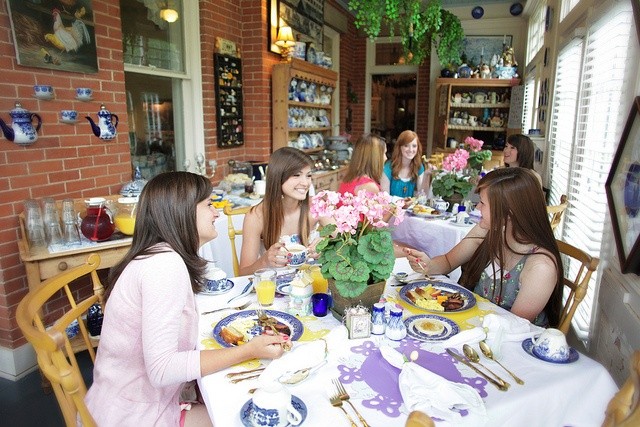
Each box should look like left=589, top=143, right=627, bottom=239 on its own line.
left=285, top=245, right=310, bottom=267
left=202, top=268, right=226, bottom=289
left=311, top=293, right=331, bottom=317
left=531, top=328, right=571, bottom=361
left=308, top=265, right=328, bottom=293
left=254, top=267, right=277, bottom=306
left=450, top=118, right=467, bottom=125
left=251, top=385, right=302, bottom=427
left=22, top=198, right=81, bottom=247
left=456, top=211, right=470, bottom=223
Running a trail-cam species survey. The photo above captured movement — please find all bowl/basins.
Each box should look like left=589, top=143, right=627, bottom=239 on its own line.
left=76, top=87, right=93, bottom=100
left=33, top=85, right=54, bottom=97
left=60, top=109, right=79, bottom=119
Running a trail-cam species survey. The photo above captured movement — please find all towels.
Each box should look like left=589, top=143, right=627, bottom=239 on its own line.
left=442, top=311, right=539, bottom=347
left=260, top=323, right=365, bottom=379
left=381, top=343, right=487, bottom=423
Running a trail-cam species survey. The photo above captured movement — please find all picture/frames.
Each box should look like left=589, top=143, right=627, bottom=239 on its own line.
left=267, top=0, right=324, bottom=55
left=464, top=34, right=513, bottom=65
left=605, top=97, right=639, bottom=275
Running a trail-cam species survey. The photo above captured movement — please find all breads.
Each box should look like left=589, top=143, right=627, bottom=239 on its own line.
left=220, top=326, right=244, bottom=346
left=406, top=410, right=436, bottom=427
left=405, top=283, right=432, bottom=302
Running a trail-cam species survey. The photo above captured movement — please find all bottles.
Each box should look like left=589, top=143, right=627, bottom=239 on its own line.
left=386, top=308, right=407, bottom=340
left=245, top=178, right=254, bottom=196
left=371, top=302, right=387, bottom=334
left=82, top=197, right=116, bottom=242
left=116, top=198, right=139, bottom=235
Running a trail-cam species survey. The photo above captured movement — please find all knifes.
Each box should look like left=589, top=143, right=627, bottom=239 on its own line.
left=445, top=348, right=508, bottom=392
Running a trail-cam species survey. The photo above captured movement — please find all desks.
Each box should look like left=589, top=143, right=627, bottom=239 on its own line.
left=17, top=196, right=134, bottom=394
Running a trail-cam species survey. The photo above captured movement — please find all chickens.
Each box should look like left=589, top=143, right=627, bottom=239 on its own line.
left=43, top=26, right=72, bottom=56
left=50, top=7, right=91, bottom=62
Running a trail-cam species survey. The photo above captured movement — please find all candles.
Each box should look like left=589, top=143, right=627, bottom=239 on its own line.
left=504, top=32, right=506, bottom=44
left=481, top=46, right=484, bottom=55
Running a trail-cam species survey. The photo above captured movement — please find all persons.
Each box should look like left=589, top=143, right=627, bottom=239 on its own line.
left=378, top=130, right=438, bottom=201
left=237, top=145, right=341, bottom=277
left=335, top=132, right=415, bottom=229
left=497, top=42, right=519, bottom=68
left=401, top=165, right=565, bottom=330
left=467, top=133, right=544, bottom=193
left=75, top=170, right=294, bottom=426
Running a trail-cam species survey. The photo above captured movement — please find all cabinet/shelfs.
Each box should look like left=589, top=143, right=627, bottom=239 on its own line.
left=312, top=171, right=337, bottom=194
left=436, top=149, right=505, bottom=169
left=214, top=52, right=243, bottom=147
left=337, top=164, right=349, bottom=180
left=434, top=78, right=521, bottom=153
left=271, top=57, right=338, bottom=153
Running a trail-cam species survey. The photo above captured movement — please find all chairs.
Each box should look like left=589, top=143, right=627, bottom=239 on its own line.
left=224, top=206, right=253, bottom=278
left=15, top=254, right=105, bottom=427
left=556, top=239, right=599, bottom=336
left=547, top=195, right=568, bottom=232
left=432, top=152, right=444, bottom=166
left=422, top=155, right=436, bottom=171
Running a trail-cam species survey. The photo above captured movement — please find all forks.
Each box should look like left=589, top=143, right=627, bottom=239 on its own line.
left=202, top=300, right=251, bottom=316
left=331, top=378, right=370, bottom=426
left=252, top=302, right=292, bottom=352
left=327, top=392, right=359, bottom=426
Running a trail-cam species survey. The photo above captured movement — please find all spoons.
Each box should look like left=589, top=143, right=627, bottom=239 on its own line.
left=479, top=342, right=525, bottom=385
left=463, top=344, right=511, bottom=388
left=228, top=281, right=253, bottom=303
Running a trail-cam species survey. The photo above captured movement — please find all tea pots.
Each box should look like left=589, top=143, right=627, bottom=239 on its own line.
left=467, top=92, right=487, bottom=104
left=468, top=116, right=478, bottom=125
left=0, top=102, right=42, bottom=144
left=85, top=105, right=120, bottom=141
left=297, top=134, right=310, bottom=150
left=487, top=92, right=499, bottom=104
left=487, top=116, right=505, bottom=128
left=309, top=133, right=324, bottom=150
left=450, top=93, right=464, bottom=103
left=432, top=196, right=450, bottom=212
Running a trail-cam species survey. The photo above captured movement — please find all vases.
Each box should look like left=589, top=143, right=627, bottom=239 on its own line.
left=442, top=194, right=463, bottom=212
left=474, top=164, right=484, bottom=175
left=327, top=276, right=385, bottom=322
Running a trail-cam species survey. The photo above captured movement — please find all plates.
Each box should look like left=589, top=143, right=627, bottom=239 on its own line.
left=406, top=205, right=437, bottom=218
left=239, top=394, right=308, bottom=427
left=275, top=274, right=296, bottom=294
left=522, top=339, right=579, bottom=363
left=447, top=219, right=476, bottom=228
left=213, top=309, right=303, bottom=348
left=198, top=279, right=234, bottom=296
left=403, top=315, right=459, bottom=341
left=399, top=282, right=477, bottom=312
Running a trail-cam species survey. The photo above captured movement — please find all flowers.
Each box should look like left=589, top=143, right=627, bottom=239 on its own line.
left=312, top=190, right=405, bottom=298
left=432, top=149, right=473, bottom=196
left=341, top=131, right=352, bottom=145
left=464, top=137, right=492, bottom=167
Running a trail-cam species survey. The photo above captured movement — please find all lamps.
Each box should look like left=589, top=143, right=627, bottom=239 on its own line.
left=182, top=152, right=216, bottom=179
left=277, top=27, right=294, bottom=63
